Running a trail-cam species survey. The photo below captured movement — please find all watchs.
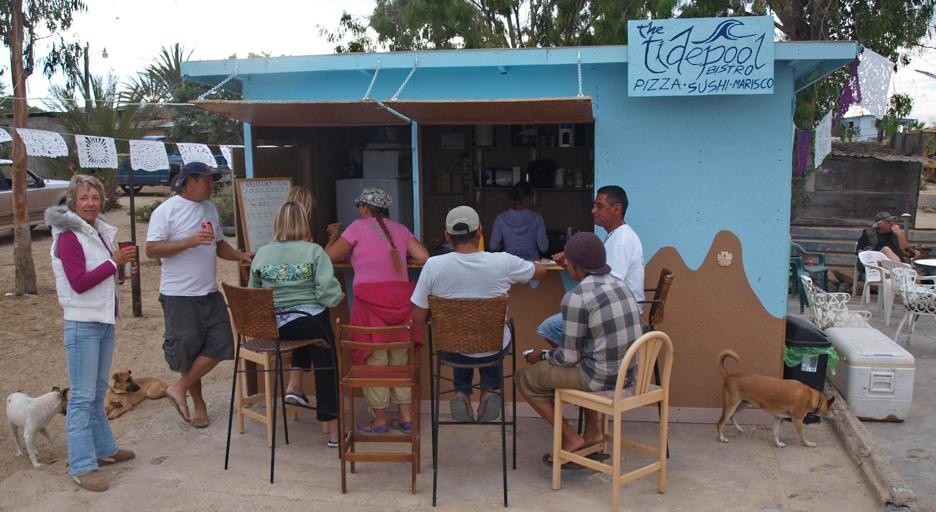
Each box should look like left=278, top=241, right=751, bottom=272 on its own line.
left=540, top=350, right=551, bottom=361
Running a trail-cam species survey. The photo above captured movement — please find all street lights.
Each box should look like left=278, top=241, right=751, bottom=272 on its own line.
left=84, top=42, right=108, bottom=111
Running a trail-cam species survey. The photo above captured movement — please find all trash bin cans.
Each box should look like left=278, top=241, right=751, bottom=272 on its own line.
left=784, top=314, right=831, bottom=424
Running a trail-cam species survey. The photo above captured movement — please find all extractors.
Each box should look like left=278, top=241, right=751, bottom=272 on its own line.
left=473, top=125, right=543, bottom=168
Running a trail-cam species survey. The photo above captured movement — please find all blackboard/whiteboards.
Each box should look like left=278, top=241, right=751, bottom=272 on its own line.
left=234, top=176, right=293, bottom=252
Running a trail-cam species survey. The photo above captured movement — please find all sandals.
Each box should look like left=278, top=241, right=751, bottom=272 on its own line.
left=354, top=420, right=389, bottom=434
left=389, top=418, right=412, bottom=433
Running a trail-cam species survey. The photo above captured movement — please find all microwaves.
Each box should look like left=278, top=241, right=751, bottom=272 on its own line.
left=362, top=143, right=412, bottom=180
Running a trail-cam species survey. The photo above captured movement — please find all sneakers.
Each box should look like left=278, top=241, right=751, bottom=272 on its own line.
left=97, top=448, right=135, bottom=466
left=71, top=469, right=109, bottom=492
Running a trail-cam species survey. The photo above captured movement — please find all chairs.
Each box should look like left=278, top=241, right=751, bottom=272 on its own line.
left=892, top=267, right=935, bottom=343
left=790, top=242, right=830, bottom=302
left=332, top=316, right=422, bottom=496
left=552, top=328, right=675, bottom=512
left=879, top=259, right=915, bottom=329
left=217, top=284, right=300, bottom=448
left=789, top=257, right=809, bottom=313
left=799, top=274, right=873, bottom=330
left=858, top=250, right=912, bottom=313
left=220, top=281, right=340, bottom=485
left=424, top=293, right=518, bottom=509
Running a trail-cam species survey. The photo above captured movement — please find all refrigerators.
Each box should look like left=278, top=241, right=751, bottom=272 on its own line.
left=334, top=178, right=419, bottom=317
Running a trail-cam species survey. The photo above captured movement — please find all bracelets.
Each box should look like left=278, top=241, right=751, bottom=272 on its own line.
left=106, top=257, right=119, bottom=271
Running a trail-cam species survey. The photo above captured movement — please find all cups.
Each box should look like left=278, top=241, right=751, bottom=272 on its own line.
left=117, top=241, right=136, bottom=281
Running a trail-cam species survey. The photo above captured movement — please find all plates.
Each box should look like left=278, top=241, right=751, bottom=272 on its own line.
left=533, top=259, right=556, bottom=267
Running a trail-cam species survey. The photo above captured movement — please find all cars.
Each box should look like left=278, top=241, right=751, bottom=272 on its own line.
left=0, top=159, right=70, bottom=232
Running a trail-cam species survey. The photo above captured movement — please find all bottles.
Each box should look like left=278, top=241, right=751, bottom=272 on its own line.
left=566, top=226, right=572, bottom=241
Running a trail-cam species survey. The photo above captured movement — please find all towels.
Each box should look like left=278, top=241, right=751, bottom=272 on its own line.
left=794, top=129, right=813, bottom=175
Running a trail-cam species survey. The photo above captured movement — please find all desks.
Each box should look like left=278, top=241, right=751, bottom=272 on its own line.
left=914, top=258, right=936, bottom=267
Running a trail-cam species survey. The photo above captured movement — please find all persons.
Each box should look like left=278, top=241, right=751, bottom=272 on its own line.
left=146, top=161, right=256, bottom=429
left=247, top=201, right=350, bottom=448
left=514, top=232, right=643, bottom=470
left=287, top=184, right=315, bottom=222
left=48, top=174, right=137, bottom=493
left=323, top=187, right=431, bottom=435
left=857, top=211, right=910, bottom=281
left=537, top=185, right=647, bottom=349
left=489, top=182, right=548, bottom=261
left=410, top=205, right=547, bottom=423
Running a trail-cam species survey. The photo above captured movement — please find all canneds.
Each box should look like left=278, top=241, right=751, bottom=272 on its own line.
left=201, top=220, right=213, bottom=234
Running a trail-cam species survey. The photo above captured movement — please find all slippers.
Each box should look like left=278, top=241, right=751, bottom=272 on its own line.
left=191, top=415, right=210, bottom=428
left=476, top=394, right=500, bottom=421
left=284, top=392, right=310, bottom=406
left=584, top=451, right=611, bottom=461
left=542, top=453, right=586, bottom=470
left=328, top=432, right=350, bottom=447
left=449, top=395, right=476, bottom=422
left=165, top=393, right=191, bottom=423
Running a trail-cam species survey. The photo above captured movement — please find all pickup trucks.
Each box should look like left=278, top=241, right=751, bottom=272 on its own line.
left=118, top=137, right=230, bottom=192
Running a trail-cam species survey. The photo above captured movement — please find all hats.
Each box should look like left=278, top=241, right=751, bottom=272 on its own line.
left=353, top=188, right=393, bottom=210
left=446, top=207, right=479, bottom=235
left=564, top=232, right=611, bottom=275
left=178, top=162, right=223, bottom=187
left=874, top=212, right=898, bottom=222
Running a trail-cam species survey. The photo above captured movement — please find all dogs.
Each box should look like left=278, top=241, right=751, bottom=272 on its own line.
left=5, top=386, right=70, bottom=471
left=713, top=347, right=837, bottom=449
left=102, top=368, right=191, bottom=422
left=907, top=245, right=936, bottom=281
